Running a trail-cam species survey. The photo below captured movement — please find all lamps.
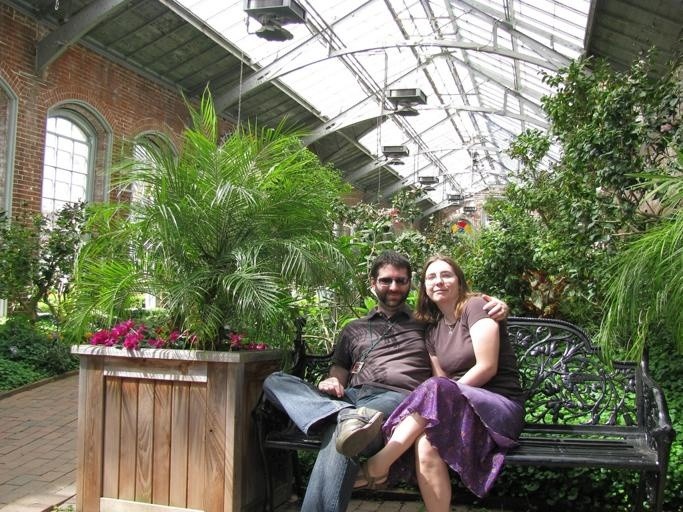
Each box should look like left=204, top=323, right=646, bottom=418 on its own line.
left=378, top=84, right=478, bottom=216
left=241, top=1, right=307, bottom=42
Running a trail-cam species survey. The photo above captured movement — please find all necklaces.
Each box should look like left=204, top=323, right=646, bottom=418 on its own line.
left=352, top=315, right=399, bottom=374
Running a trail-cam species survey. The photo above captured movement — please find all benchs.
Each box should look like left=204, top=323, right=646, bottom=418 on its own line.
left=249, top=316, right=676, bottom=512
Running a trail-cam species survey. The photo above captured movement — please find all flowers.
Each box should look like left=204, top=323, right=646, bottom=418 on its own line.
left=82, top=317, right=266, bottom=353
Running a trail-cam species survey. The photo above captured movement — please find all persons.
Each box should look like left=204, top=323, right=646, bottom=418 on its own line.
left=352, top=256, right=524, bottom=512
left=263, top=252, right=508, bottom=512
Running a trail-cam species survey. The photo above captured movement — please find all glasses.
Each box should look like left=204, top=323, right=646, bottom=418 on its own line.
left=377, top=278, right=410, bottom=286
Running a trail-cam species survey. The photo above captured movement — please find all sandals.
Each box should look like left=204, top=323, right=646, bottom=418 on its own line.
left=350, top=458, right=389, bottom=490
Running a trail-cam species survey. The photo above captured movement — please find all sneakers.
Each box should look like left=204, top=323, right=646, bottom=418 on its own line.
left=335, top=407, right=385, bottom=458
